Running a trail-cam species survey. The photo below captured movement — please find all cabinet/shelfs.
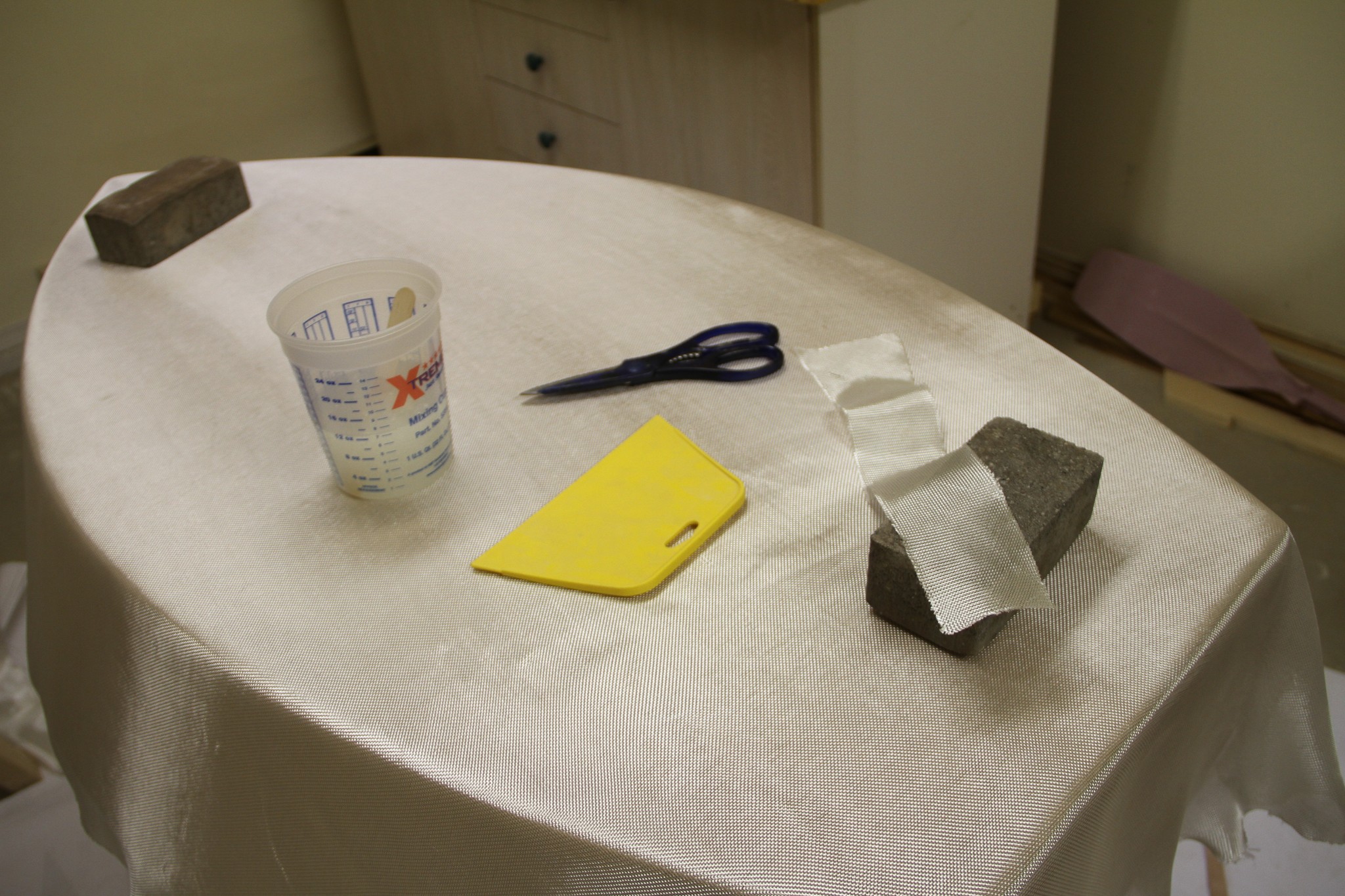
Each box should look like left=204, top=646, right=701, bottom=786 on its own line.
left=481, top=1, right=812, bottom=222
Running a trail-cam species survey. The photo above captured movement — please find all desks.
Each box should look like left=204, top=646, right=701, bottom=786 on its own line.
left=20, top=149, right=1292, bottom=896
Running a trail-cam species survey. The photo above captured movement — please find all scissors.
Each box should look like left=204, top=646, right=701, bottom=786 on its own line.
left=517, top=320, right=786, bottom=401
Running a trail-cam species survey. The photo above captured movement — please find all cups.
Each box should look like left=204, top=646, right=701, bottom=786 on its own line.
left=265, top=254, right=454, bottom=498
